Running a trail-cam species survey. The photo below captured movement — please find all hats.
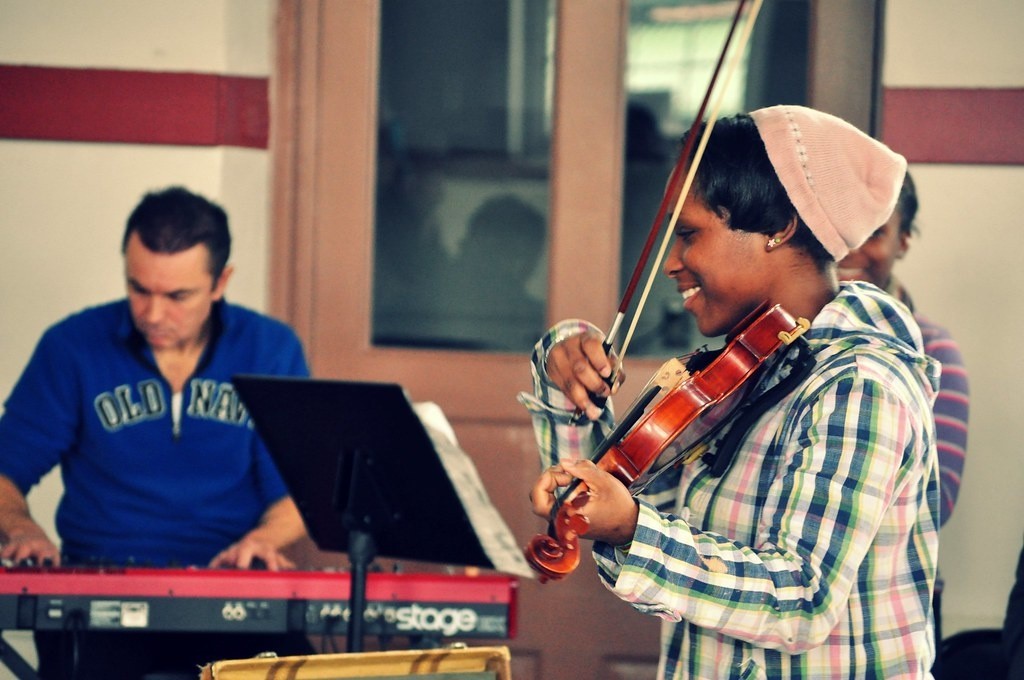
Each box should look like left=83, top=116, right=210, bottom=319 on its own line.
left=748, top=104, right=910, bottom=262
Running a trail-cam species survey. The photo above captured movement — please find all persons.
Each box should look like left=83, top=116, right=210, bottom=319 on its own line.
left=519, top=104, right=971, bottom=680
left=0, top=188, right=314, bottom=573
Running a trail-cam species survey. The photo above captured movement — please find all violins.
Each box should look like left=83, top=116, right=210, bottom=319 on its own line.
left=520, top=299, right=803, bottom=579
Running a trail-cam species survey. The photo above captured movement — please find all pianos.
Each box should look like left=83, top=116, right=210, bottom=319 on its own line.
left=0, top=563, right=518, bottom=680
